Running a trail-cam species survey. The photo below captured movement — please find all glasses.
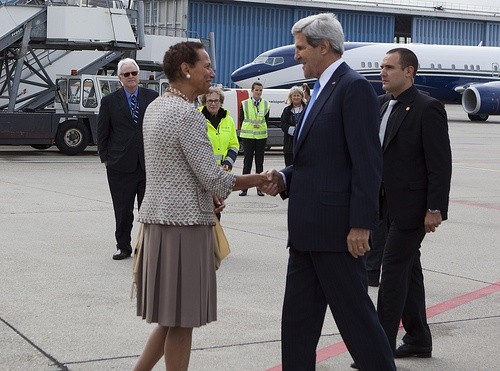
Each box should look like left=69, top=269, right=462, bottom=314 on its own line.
left=206, top=98, right=222, bottom=104
left=120, top=71, right=138, bottom=77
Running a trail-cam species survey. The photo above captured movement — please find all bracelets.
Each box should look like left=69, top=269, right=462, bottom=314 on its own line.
left=427, top=208, right=441, bottom=213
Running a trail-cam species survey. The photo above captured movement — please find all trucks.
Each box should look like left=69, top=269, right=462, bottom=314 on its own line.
left=0, top=70, right=125, bottom=156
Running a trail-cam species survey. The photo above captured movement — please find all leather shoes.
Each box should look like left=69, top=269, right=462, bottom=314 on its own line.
left=394, top=341, right=432, bottom=358
left=113, top=249, right=132, bottom=259
left=351, top=363, right=358, bottom=369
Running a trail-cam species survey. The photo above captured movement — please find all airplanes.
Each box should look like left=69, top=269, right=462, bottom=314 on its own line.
left=230, top=39, right=500, bottom=121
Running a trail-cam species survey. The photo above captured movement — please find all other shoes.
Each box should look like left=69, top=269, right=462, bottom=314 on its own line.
left=257, top=190, right=264, bottom=196
left=239, top=190, right=247, bottom=196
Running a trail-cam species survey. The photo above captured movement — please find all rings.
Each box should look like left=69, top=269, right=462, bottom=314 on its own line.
left=358, top=246, right=364, bottom=251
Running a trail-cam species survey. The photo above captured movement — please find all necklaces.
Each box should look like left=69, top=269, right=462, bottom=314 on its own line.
left=166, top=86, right=190, bottom=102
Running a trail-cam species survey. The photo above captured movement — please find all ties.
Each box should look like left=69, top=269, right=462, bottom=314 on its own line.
left=129, top=95, right=140, bottom=124
left=297, top=81, right=321, bottom=143
left=379, top=100, right=398, bottom=150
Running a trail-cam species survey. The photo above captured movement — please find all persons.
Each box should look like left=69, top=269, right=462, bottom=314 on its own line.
left=131, top=41, right=269, bottom=371
left=300, top=82, right=311, bottom=102
left=197, top=88, right=239, bottom=223
left=350, top=48, right=452, bottom=371
left=97, top=58, right=160, bottom=261
left=281, top=86, right=307, bottom=168
left=239, top=82, right=270, bottom=197
left=258, top=12, right=399, bottom=371
